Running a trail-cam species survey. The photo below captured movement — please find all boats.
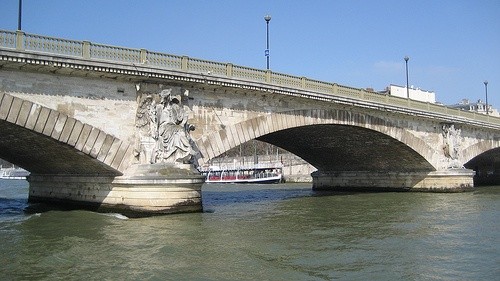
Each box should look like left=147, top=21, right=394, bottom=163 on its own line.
left=199, top=162, right=283, bottom=183
left=0, top=165, right=30, bottom=179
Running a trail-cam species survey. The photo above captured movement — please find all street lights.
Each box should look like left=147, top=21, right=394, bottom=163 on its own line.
left=404, top=56, right=410, bottom=98
left=264, top=15, right=272, bottom=69
left=483, top=81, right=488, bottom=114
